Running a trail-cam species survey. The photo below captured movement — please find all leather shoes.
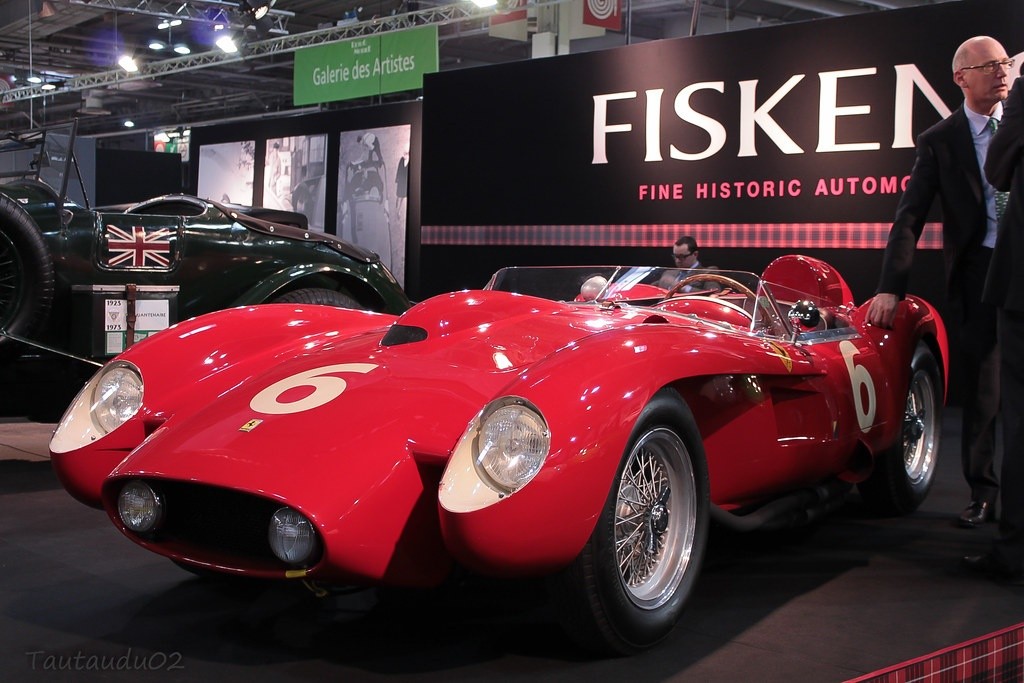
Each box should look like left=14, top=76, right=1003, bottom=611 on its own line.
left=961, top=501, right=995, bottom=528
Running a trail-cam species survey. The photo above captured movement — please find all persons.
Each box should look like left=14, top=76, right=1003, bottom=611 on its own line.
left=866, top=35, right=1011, bottom=527
left=266, top=141, right=281, bottom=194
left=393, top=143, right=409, bottom=218
left=352, top=132, right=389, bottom=210
left=962, top=65, right=1022, bottom=586
left=647, top=236, right=721, bottom=293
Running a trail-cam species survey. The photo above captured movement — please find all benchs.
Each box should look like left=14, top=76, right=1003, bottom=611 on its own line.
left=715, top=297, right=827, bottom=330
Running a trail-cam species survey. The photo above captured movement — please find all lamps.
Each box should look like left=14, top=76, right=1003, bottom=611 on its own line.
left=148, top=17, right=190, bottom=55
left=238, top=0, right=274, bottom=36
left=154, top=128, right=190, bottom=142
left=155, top=16, right=181, bottom=29
left=208, top=25, right=224, bottom=31
left=42, top=80, right=70, bottom=91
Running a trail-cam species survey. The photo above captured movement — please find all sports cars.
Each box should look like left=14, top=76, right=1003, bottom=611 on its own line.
left=0, top=174, right=414, bottom=391
left=46, top=254, right=951, bottom=654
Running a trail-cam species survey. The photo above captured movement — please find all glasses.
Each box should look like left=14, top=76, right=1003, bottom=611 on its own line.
left=960, top=58, right=1015, bottom=73
left=671, top=253, right=691, bottom=260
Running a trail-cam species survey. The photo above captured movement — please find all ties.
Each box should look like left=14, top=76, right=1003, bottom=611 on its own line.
left=988, top=118, right=1010, bottom=225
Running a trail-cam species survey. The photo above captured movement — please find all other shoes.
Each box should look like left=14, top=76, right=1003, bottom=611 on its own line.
left=964, top=553, right=1024, bottom=580
